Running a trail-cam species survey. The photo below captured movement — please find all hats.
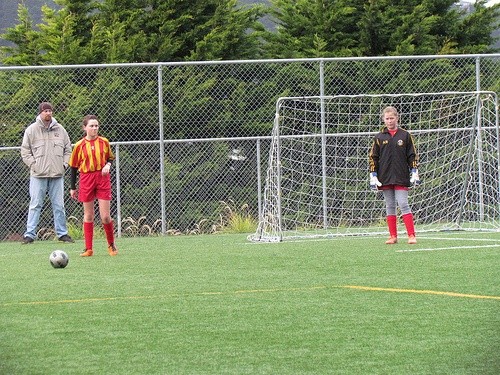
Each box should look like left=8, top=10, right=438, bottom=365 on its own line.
left=37, top=102, right=52, bottom=115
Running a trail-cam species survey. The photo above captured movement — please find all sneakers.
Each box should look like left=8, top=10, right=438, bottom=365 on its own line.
left=80, top=248, right=93, bottom=257
left=108, top=243, right=118, bottom=256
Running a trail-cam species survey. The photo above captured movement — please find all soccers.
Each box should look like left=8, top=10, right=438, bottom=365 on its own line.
left=49, top=250, right=69, bottom=268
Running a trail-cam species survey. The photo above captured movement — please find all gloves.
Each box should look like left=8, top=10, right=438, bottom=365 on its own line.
left=370, top=172, right=382, bottom=186
left=410, top=168, right=421, bottom=187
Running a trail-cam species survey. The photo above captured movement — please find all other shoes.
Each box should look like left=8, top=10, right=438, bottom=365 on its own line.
left=58, top=234, right=75, bottom=243
left=21, top=236, right=33, bottom=244
left=408, top=237, right=416, bottom=244
left=385, top=237, right=397, bottom=244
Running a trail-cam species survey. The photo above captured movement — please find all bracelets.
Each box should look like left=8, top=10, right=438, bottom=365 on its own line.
left=107, top=163, right=111, bottom=166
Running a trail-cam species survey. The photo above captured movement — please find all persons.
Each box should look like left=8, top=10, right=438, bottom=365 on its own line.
left=69, top=116, right=118, bottom=257
left=369, top=106, right=419, bottom=244
left=21, top=103, right=75, bottom=245
